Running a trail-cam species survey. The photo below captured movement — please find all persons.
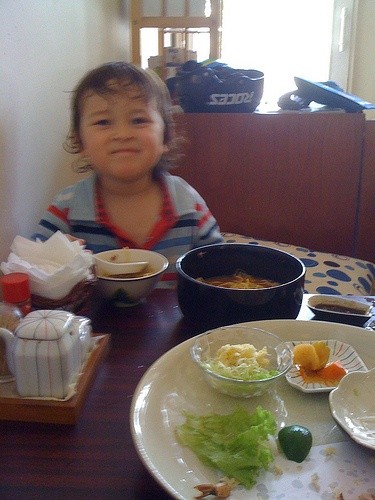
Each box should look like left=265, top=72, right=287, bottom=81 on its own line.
left=32, top=62, right=226, bottom=289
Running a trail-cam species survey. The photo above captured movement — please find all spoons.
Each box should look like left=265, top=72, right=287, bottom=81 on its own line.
left=92, top=254, right=150, bottom=275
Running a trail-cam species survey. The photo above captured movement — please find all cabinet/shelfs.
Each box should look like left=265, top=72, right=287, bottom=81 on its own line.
left=168, top=112, right=374, bottom=262
left=130, top=0, right=223, bottom=77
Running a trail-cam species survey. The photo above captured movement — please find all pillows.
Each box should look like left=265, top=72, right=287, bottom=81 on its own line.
left=222, top=231, right=372, bottom=297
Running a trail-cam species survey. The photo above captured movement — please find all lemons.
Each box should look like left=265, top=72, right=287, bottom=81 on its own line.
left=278, top=424, right=313, bottom=463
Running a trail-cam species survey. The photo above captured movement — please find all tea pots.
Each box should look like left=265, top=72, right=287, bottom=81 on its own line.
left=0, top=327, right=16, bottom=376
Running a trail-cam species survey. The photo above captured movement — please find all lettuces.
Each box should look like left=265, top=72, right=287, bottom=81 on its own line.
left=205, top=359, right=281, bottom=396
left=174, top=404, right=278, bottom=487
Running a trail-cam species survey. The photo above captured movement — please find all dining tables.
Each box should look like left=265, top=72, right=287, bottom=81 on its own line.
left=0, top=256, right=374, bottom=500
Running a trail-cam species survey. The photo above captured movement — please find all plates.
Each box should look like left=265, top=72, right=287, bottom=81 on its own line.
left=328, top=369, right=375, bottom=449
left=130, top=319, right=375, bottom=500
left=274, top=339, right=368, bottom=394
left=306, top=295, right=372, bottom=320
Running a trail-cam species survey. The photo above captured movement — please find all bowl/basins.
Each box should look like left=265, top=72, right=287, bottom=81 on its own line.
left=90, top=249, right=169, bottom=307
left=176, top=243, right=305, bottom=331
left=191, top=327, right=294, bottom=398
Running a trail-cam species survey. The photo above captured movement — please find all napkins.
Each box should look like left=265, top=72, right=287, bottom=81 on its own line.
left=0, top=227, right=97, bottom=299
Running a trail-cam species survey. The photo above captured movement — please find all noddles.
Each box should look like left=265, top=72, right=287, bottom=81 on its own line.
left=196, top=273, right=281, bottom=289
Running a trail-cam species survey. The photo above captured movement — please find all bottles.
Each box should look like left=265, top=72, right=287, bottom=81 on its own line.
left=14, top=309, right=86, bottom=399
left=0, top=273, right=32, bottom=316
